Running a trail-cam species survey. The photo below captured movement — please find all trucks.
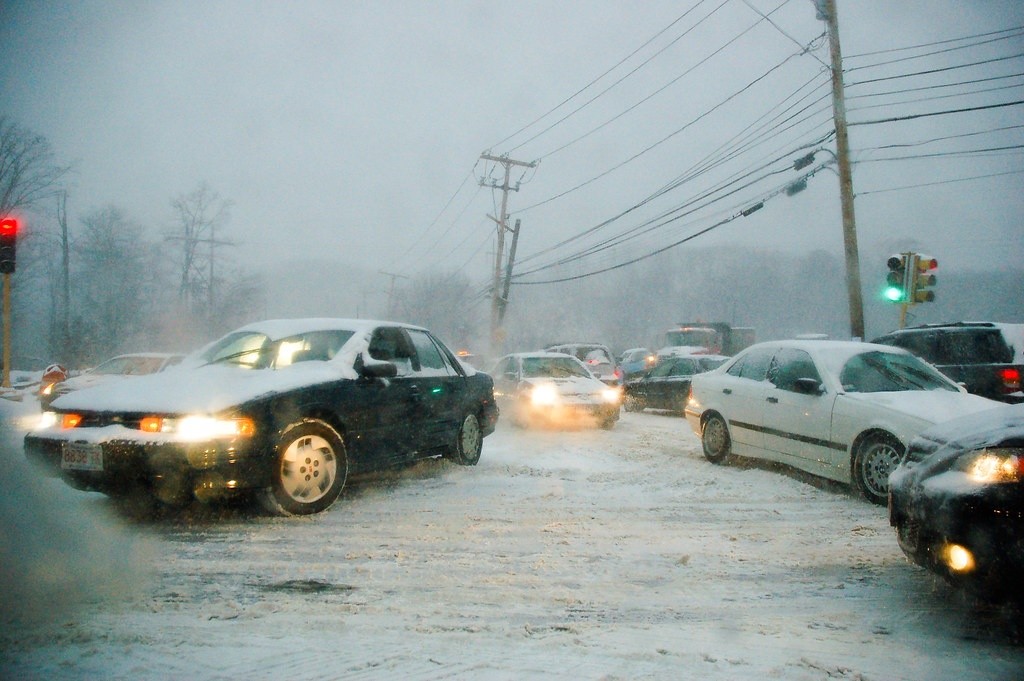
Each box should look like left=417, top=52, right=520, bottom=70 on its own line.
left=664, top=321, right=758, bottom=358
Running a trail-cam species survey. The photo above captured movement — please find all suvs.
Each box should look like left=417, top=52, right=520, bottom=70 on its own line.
left=543, top=342, right=620, bottom=389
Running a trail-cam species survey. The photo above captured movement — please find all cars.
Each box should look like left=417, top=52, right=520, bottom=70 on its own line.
left=888, top=399, right=1024, bottom=607
left=684, top=339, right=1013, bottom=510
left=23, top=316, right=499, bottom=524
left=619, top=344, right=711, bottom=382
left=485, top=352, right=621, bottom=431
left=40, top=351, right=188, bottom=413
left=622, top=354, right=731, bottom=412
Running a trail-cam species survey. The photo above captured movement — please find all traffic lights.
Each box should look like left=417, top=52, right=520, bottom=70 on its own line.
left=910, top=252, right=940, bottom=305
left=0, top=216, right=17, bottom=276
left=884, top=251, right=906, bottom=303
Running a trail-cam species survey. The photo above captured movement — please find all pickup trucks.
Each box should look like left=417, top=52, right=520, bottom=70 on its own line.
left=869, top=322, right=1024, bottom=406
